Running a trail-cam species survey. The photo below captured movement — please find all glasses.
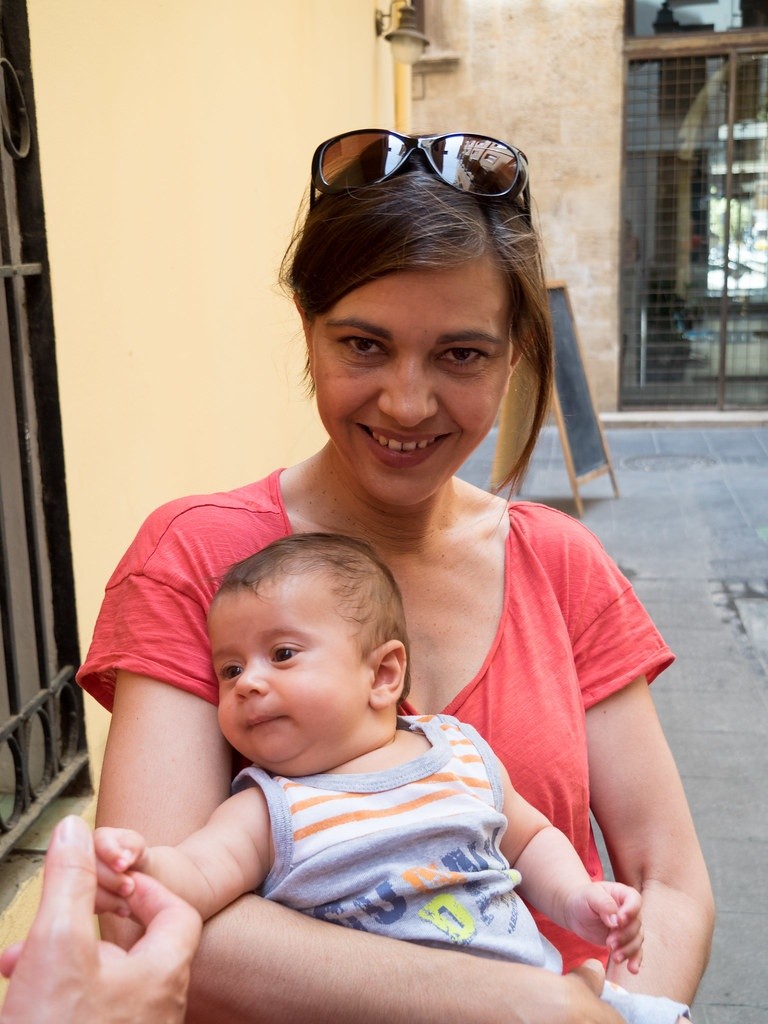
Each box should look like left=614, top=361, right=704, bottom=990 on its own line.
left=308, top=129, right=531, bottom=228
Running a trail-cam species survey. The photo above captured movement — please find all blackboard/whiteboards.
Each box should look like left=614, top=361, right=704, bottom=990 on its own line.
left=543, top=281, right=610, bottom=486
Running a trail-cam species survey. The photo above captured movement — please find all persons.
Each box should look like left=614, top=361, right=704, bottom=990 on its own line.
left=93, top=532, right=694, bottom=1024
left=0, top=813, right=202, bottom=1024
left=75, top=132, right=714, bottom=1024
